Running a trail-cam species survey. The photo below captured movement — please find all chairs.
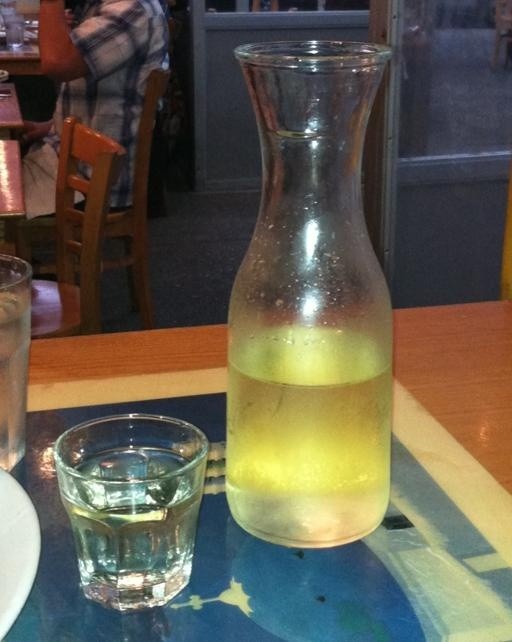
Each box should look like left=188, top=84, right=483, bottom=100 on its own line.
left=31, top=66, right=174, bottom=339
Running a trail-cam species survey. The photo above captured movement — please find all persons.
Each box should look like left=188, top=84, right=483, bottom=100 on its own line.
left=14, top=0, right=173, bottom=221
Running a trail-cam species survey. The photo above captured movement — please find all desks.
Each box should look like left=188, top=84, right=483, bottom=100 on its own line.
left=0, top=41, right=40, bottom=219
left=0, top=297, right=512, bottom=642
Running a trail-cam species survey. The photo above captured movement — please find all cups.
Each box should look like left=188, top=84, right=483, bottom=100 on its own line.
left=52, top=412, right=211, bottom=612
left=0, top=251, right=33, bottom=472
left=0, top=1, right=25, bottom=48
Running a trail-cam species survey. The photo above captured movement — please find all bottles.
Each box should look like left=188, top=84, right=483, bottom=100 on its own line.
left=223, top=40, right=393, bottom=551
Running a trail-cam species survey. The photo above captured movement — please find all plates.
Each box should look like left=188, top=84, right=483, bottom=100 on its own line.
left=0, top=466, right=43, bottom=641
